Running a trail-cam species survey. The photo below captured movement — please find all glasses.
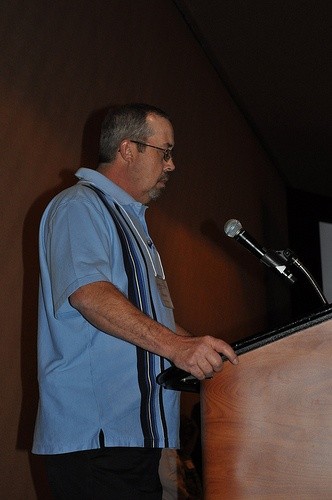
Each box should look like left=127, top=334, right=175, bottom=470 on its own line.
left=119, top=140, right=177, bottom=163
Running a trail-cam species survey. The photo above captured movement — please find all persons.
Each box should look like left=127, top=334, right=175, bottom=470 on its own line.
left=31, top=102, right=238, bottom=500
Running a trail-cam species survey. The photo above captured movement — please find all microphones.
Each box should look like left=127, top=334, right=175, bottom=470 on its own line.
left=224, top=219, right=296, bottom=283
